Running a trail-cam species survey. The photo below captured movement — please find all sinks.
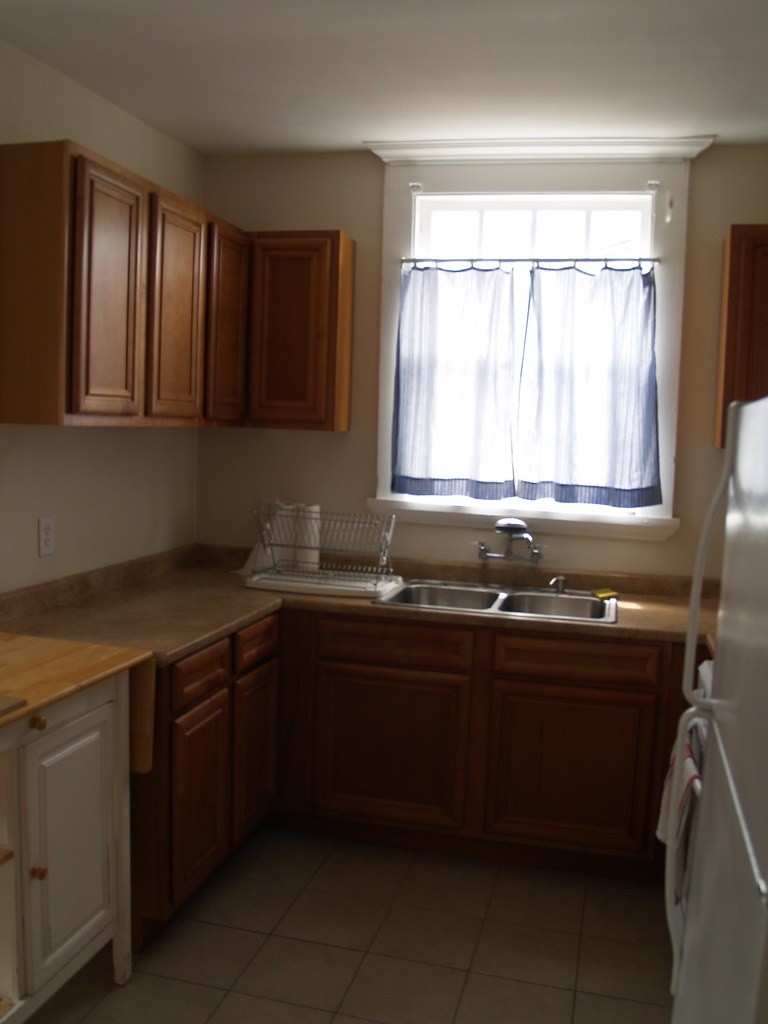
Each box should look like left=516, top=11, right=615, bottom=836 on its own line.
left=498, top=591, right=607, bottom=619
left=386, top=583, right=499, bottom=610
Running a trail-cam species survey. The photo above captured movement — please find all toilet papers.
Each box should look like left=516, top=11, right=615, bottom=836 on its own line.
left=232, top=502, right=322, bottom=575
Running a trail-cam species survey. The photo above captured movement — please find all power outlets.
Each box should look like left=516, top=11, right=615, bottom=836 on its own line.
left=38, top=516, right=56, bottom=557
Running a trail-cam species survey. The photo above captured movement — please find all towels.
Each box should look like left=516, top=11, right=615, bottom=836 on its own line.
left=655, top=740, right=703, bottom=908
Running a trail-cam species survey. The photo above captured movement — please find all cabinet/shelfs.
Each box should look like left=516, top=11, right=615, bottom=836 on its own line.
left=0, top=138, right=249, bottom=429
left=130, top=612, right=280, bottom=954
left=0, top=632, right=153, bottom=1024
left=714, top=223, right=768, bottom=450
left=280, top=608, right=684, bottom=890
left=249, top=229, right=355, bottom=433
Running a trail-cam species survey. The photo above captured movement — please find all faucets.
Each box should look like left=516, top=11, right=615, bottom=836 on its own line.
left=478, top=517, right=543, bottom=565
left=549, top=575, right=566, bottom=593
